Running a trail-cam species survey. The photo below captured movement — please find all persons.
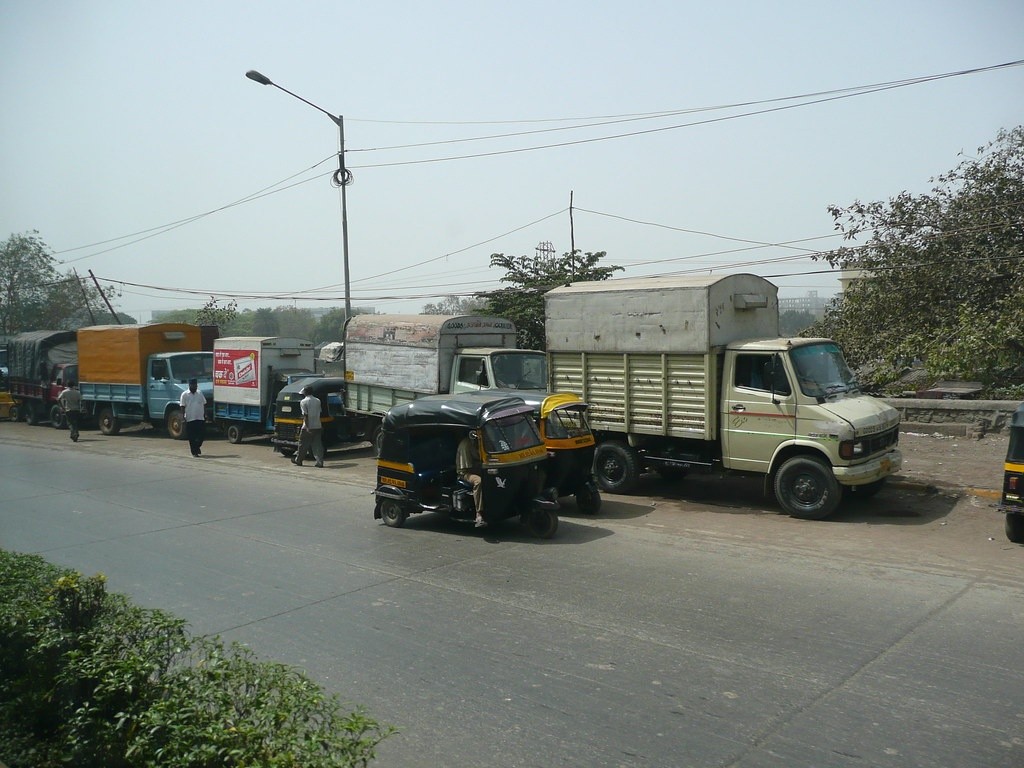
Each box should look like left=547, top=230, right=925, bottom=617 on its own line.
left=455, top=427, right=490, bottom=527
left=291, top=385, right=324, bottom=467
left=180, top=377, right=208, bottom=456
left=57, top=380, right=81, bottom=442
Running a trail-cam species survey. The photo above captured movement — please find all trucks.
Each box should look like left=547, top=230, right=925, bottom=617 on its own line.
left=76, top=320, right=214, bottom=441
left=8, top=329, right=78, bottom=429
left=341, top=314, right=550, bottom=459
left=211, top=337, right=326, bottom=444
left=544, top=272, right=902, bottom=522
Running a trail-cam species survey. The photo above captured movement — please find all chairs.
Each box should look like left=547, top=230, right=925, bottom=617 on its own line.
left=447, top=442, right=468, bottom=488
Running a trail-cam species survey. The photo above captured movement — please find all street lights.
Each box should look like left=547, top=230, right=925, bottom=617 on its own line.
left=245, top=70, right=351, bottom=337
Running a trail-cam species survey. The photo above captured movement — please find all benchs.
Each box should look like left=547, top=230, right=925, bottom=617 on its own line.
left=410, top=435, right=450, bottom=490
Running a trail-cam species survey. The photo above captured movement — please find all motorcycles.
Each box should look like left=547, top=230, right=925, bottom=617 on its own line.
left=370, top=391, right=602, bottom=538
left=0, top=367, right=23, bottom=422
left=998, top=398, right=1024, bottom=542
left=268, top=374, right=345, bottom=459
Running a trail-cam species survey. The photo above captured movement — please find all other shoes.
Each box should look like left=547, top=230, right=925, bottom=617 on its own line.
left=474, top=519, right=488, bottom=527
left=291, top=456, right=302, bottom=466
left=194, top=454, right=199, bottom=458
left=198, top=449, right=201, bottom=454
left=314, top=464, right=323, bottom=468
